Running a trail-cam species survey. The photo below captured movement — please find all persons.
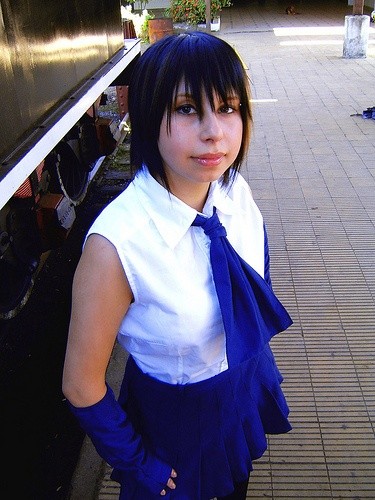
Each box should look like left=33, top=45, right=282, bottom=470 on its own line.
left=62, top=31, right=294, bottom=500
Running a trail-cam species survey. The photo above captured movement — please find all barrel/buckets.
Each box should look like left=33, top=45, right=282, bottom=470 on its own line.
left=147, top=18, right=174, bottom=46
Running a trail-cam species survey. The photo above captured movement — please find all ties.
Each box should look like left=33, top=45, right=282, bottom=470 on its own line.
left=191, top=206, right=294, bottom=370
